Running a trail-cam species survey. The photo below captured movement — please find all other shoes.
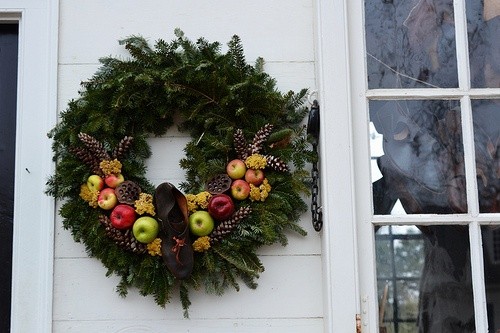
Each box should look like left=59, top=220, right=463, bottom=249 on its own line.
left=152, top=177, right=194, bottom=280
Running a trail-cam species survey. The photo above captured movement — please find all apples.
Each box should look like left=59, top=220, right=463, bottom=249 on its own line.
left=98, top=188, right=118, bottom=211
left=245, top=168, right=264, bottom=187
left=132, top=216, right=159, bottom=244
left=231, top=179, right=251, bottom=200
left=104, top=173, right=125, bottom=188
left=87, top=175, right=104, bottom=192
left=109, top=204, right=137, bottom=231
left=189, top=211, right=215, bottom=237
left=226, top=159, right=246, bottom=180
left=207, top=194, right=235, bottom=221
